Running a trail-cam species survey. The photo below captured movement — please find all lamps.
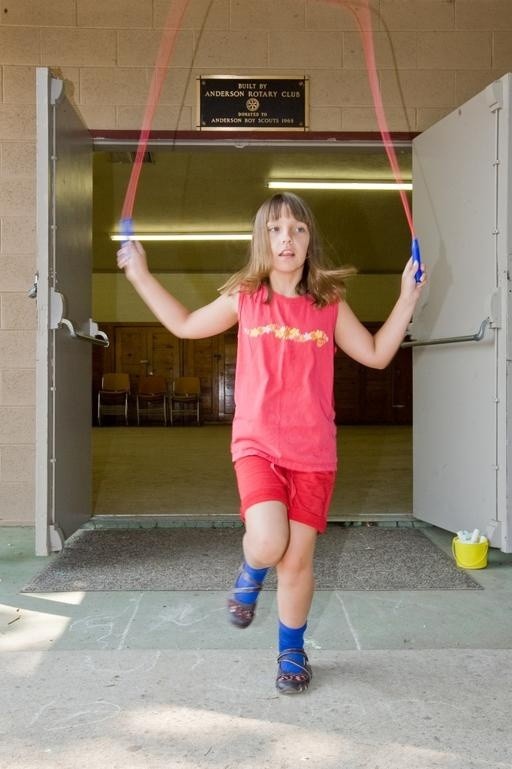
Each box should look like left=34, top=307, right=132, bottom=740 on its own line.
left=266, top=176, right=412, bottom=192
left=108, top=229, right=252, bottom=242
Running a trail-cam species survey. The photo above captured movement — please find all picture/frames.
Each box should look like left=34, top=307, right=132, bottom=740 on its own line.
left=195, top=73, right=311, bottom=133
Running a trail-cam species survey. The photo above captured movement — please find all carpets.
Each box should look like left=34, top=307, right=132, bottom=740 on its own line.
left=21, top=523, right=486, bottom=597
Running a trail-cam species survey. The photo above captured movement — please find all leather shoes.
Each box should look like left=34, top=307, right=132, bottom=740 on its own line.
left=222, top=560, right=262, bottom=629
left=274, top=647, right=315, bottom=698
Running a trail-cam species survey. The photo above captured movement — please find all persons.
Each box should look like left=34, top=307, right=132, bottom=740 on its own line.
left=114, top=187, right=429, bottom=699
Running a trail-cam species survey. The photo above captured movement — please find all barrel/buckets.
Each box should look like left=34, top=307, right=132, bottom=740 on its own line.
left=452, top=537, right=489, bottom=571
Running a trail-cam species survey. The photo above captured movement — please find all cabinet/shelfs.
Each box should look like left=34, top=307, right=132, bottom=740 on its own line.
left=88, top=321, right=238, bottom=427
left=333, top=322, right=412, bottom=426
